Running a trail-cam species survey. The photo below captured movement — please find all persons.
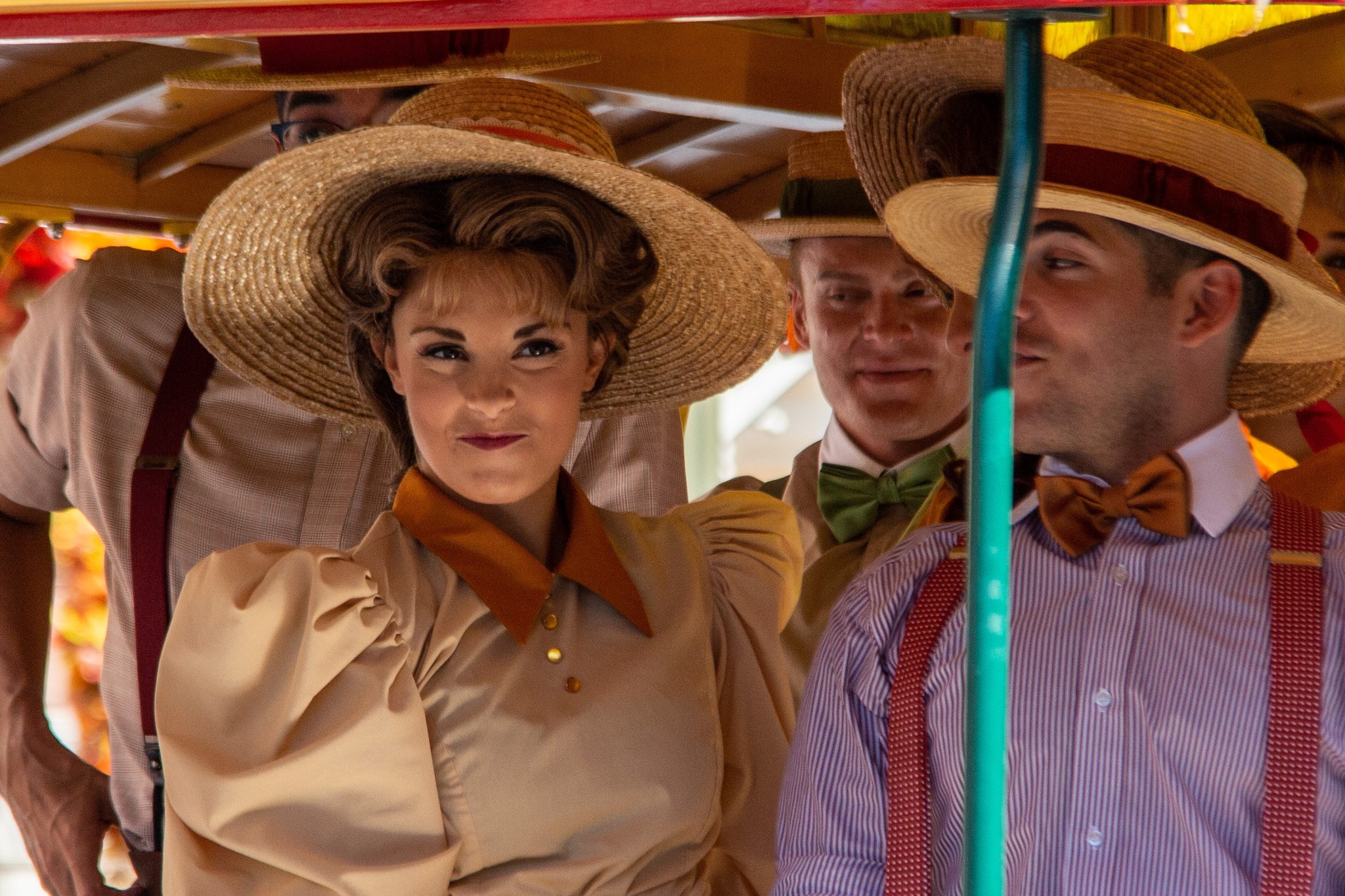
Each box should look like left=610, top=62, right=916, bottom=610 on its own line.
left=1237, top=96, right=1345, bottom=511
left=154, top=77, right=807, bottom=896
left=0, top=33, right=685, bottom=896
left=711, top=134, right=977, bottom=721
left=775, top=34, right=1345, bottom=896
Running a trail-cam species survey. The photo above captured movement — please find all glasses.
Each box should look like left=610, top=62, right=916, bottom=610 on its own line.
left=268, top=119, right=349, bottom=155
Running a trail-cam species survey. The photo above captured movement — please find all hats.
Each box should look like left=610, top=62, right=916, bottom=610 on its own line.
left=736, top=130, right=894, bottom=245
left=839, top=32, right=1345, bottom=419
left=182, top=78, right=787, bottom=424
left=883, top=90, right=1345, bottom=365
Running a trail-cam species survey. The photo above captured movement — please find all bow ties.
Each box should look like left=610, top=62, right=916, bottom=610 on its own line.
left=1034, top=454, right=1189, bottom=558
left=815, top=444, right=955, bottom=542
